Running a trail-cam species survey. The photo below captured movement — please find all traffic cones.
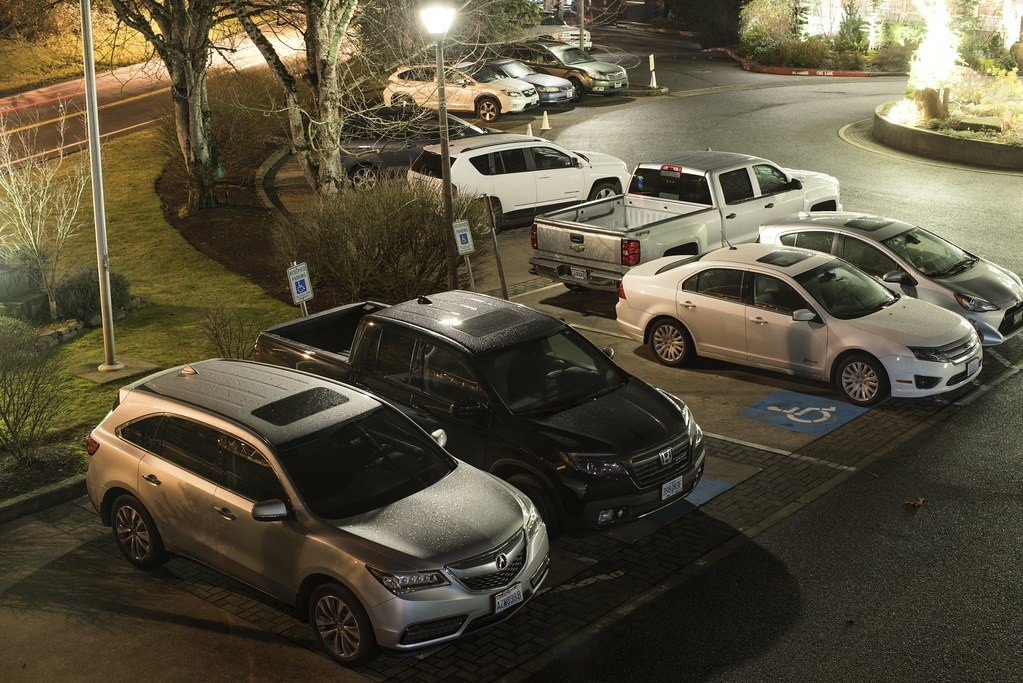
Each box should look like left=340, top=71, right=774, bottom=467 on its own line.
left=526, top=123, right=533, bottom=136
left=540, top=111, right=553, bottom=130
left=649, top=71, right=657, bottom=89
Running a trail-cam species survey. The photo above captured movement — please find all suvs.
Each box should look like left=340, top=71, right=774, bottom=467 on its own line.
left=406, top=132, right=631, bottom=241
left=499, top=39, right=629, bottom=102
left=381, top=59, right=538, bottom=122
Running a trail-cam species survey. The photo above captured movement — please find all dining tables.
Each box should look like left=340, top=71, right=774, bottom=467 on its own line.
left=286, top=420, right=410, bottom=513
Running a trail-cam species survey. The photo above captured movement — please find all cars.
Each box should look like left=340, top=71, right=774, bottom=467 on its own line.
left=481, top=57, right=577, bottom=106
left=756, top=212, right=1023, bottom=347
left=615, top=243, right=984, bottom=407
left=82, top=357, right=554, bottom=667
left=340, top=103, right=500, bottom=193
left=514, top=12, right=592, bottom=51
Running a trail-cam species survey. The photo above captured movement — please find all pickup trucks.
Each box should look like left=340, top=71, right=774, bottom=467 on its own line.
left=529, top=149, right=843, bottom=293
left=247, top=289, right=707, bottom=529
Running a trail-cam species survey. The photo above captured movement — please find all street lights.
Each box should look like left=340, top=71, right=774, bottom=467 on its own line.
left=418, top=0, right=460, bottom=289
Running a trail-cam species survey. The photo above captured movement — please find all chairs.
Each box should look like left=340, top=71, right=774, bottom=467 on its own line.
left=488, top=339, right=572, bottom=412
